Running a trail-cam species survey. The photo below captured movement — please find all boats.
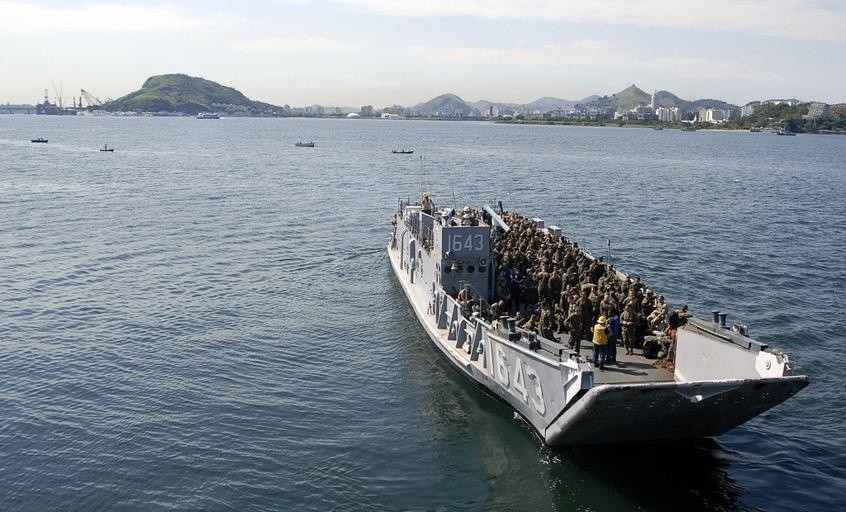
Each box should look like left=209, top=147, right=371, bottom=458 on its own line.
left=196, top=113, right=219, bottom=119
left=680, top=127, right=696, bottom=132
left=653, top=127, right=662, bottom=130
left=750, top=127, right=761, bottom=132
left=31, top=137, right=48, bottom=143
left=100, top=144, right=115, bottom=152
left=387, top=196, right=810, bottom=449
left=776, top=126, right=796, bottom=136
left=295, top=140, right=314, bottom=146
left=392, top=149, right=414, bottom=153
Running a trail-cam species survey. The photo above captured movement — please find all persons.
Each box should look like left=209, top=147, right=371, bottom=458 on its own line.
left=422, top=237, right=428, bottom=249
left=457, top=235, right=694, bottom=373
left=470, top=210, right=556, bottom=244
left=422, top=193, right=479, bottom=227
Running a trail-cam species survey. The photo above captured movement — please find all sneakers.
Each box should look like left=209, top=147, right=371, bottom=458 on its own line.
left=625, top=350, right=633, bottom=355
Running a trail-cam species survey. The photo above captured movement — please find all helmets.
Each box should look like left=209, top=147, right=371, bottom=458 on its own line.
left=597, top=316, right=606, bottom=324
left=423, top=192, right=429, bottom=198
